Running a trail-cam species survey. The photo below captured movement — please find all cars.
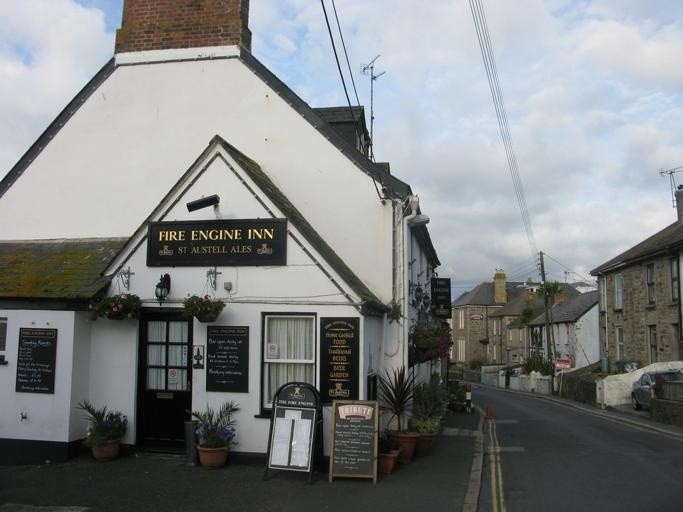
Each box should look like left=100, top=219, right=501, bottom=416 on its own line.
left=631, top=368, right=683, bottom=410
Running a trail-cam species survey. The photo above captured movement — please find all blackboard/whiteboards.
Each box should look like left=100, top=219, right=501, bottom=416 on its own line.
left=320, top=317, right=359, bottom=406
left=267, top=405, right=317, bottom=473
left=329, top=400, right=379, bottom=478
left=15, top=328, right=57, bottom=394
left=206, top=326, right=248, bottom=393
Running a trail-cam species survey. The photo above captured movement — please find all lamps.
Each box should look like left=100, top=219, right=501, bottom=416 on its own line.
left=410, top=282, right=424, bottom=307
left=155, top=273, right=170, bottom=306
left=419, top=291, right=431, bottom=310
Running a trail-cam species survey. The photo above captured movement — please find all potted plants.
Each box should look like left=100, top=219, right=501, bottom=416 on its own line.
left=383, top=428, right=403, bottom=455
left=408, top=416, right=439, bottom=456
left=378, top=436, right=398, bottom=474
left=373, top=365, right=425, bottom=465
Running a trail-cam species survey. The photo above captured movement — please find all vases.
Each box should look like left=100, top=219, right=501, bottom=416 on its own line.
left=107, top=312, right=124, bottom=320
left=195, top=314, right=218, bottom=322
left=197, top=444, right=228, bottom=470
left=92, top=439, right=121, bottom=461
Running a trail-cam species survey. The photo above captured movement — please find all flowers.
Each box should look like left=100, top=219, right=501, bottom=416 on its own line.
left=183, top=293, right=226, bottom=319
left=90, top=293, right=141, bottom=319
left=75, top=400, right=128, bottom=447
left=185, top=401, right=240, bottom=448
